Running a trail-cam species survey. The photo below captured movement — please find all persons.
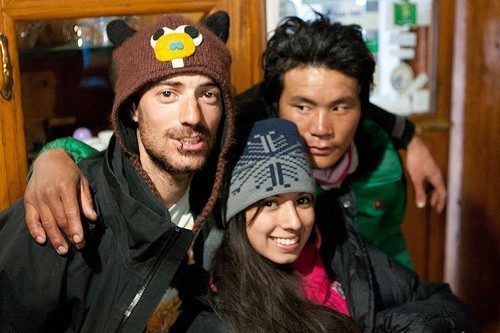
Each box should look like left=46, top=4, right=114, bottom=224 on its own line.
left=24, top=8, right=418, bottom=274
left=183, top=118, right=476, bottom=333
left=0, top=10, right=446, bottom=333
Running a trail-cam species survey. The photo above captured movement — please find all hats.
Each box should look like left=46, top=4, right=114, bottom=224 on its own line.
left=220, top=118, right=316, bottom=225
left=106, top=10, right=234, bottom=237
